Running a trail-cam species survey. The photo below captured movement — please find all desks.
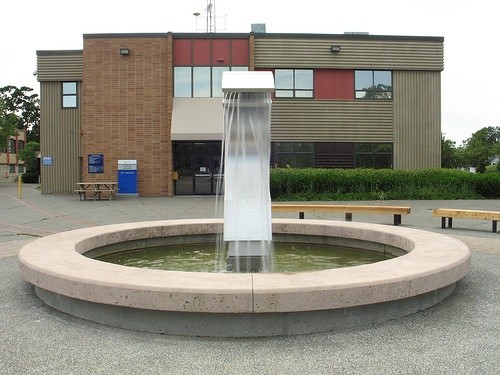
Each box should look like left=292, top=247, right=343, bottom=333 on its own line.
left=76, top=182, right=119, bottom=199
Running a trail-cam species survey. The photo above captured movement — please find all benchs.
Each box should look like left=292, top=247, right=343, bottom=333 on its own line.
left=272, top=203, right=410, bottom=226
left=434, top=208, right=500, bottom=232
left=84, top=188, right=119, bottom=198
left=75, top=189, right=117, bottom=200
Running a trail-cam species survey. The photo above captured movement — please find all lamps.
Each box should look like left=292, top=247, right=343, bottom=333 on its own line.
left=331, top=44, right=340, bottom=53
left=119, top=47, right=129, bottom=55
